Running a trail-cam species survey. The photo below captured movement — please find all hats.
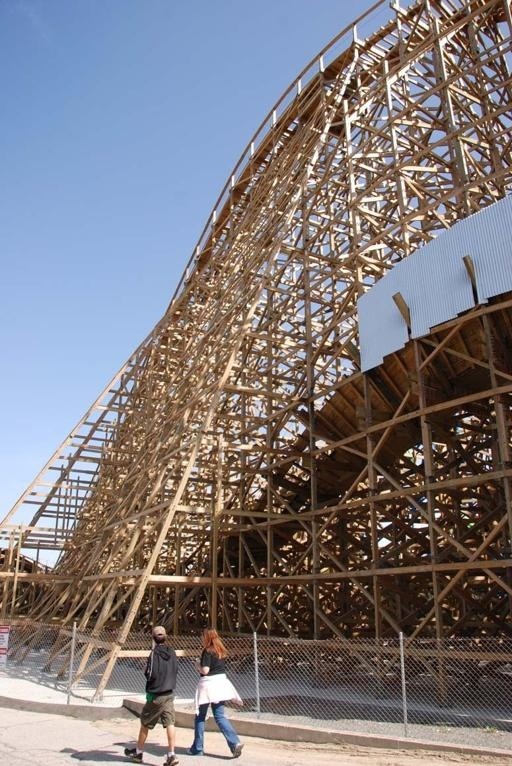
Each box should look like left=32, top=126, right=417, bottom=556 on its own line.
left=152, top=626, right=165, bottom=637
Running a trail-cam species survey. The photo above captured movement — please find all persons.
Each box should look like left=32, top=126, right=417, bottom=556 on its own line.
left=124, top=626, right=180, bottom=765
left=186, top=627, right=243, bottom=758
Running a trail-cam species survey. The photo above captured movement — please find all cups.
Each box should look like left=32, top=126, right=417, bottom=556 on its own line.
left=197, top=658, right=201, bottom=671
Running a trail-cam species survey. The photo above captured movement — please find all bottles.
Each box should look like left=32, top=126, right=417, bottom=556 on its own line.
left=146, top=693, right=153, bottom=704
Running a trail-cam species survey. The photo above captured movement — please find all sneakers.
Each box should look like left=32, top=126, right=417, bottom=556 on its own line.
left=163, top=755, right=179, bottom=766
left=233, top=743, right=244, bottom=755
left=125, top=748, right=142, bottom=762
left=186, top=748, right=203, bottom=756
